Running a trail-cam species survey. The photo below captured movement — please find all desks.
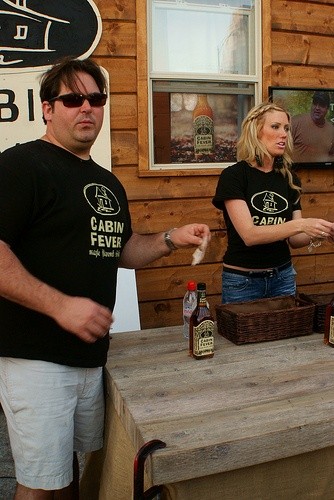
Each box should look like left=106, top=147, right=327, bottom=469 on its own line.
left=81, top=322, right=334, bottom=500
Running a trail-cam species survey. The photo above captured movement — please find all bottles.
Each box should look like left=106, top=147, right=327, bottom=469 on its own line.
left=183, top=280, right=196, bottom=339
left=193, top=93, right=216, bottom=158
left=324, top=296, right=334, bottom=348
left=192, top=295, right=210, bottom=312
left=189, top=283, right=215, bottom=360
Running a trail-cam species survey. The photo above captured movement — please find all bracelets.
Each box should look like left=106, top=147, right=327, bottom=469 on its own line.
left=164, top=227, right=177, bottom=252
left=308, top=236, right=322, bottom=252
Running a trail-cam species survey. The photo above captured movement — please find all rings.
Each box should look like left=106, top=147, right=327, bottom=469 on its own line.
left=320, top=232, right=328, bottom=237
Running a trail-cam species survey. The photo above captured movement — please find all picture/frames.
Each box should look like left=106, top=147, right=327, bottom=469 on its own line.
left=267, top=85, right=334, bottom=170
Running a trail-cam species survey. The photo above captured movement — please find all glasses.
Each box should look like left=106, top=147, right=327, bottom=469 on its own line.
left=48, top=92, right=108, bottom=108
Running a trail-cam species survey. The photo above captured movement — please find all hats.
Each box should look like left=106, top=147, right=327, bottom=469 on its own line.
left=312, top=91, right=330, bottom=105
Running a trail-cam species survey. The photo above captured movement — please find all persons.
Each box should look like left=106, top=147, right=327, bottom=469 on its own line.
left=212, top=102, right=334, bottom=304
left=290, top=92, right=334, bottom=162
left=0, top=55, right=211, bottom=500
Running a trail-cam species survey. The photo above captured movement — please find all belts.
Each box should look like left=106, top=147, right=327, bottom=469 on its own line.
left=223, top=261, right=292, bottom=278
